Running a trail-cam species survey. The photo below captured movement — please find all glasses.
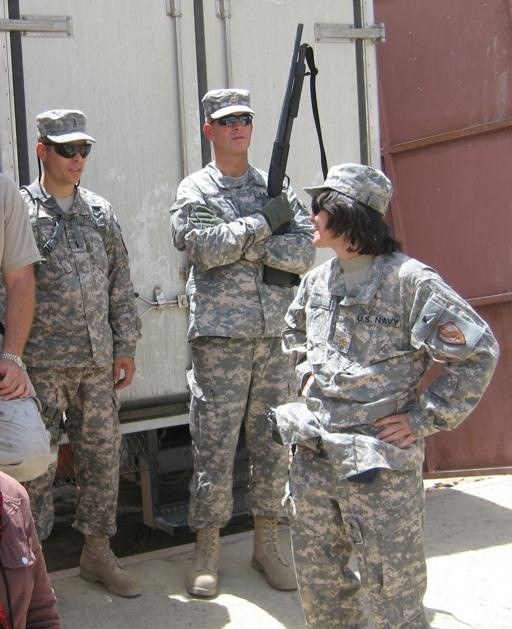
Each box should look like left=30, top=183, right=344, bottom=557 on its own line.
left=209, top=114, right=253, bottom=128
left=310, top=196, right=322, bottom=214
left=53, top=141, right=92, bottom=158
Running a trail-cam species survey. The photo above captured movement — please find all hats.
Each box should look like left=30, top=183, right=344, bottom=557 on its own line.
left=36, top=110, right=95, bottom=145
left=303, top=163, right=394, bottom=216
left=202, top=89, right=254, bottom=119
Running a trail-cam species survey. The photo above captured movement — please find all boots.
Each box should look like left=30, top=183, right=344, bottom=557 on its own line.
left=252, top=516, right=298, bottom=590
left=188, top=526, right=220, bottom=596
left=78, top=534, right=143, bottom=597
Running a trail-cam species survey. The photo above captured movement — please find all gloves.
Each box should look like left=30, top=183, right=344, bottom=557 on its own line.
left=258, top=192, right=293, bottom=231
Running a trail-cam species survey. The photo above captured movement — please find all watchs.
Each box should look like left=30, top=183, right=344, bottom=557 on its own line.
left=0, top=352, right=23, bottom=367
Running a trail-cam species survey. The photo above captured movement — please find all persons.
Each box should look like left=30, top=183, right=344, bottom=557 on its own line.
left=169, top=88, right=317, bottom=595
left=0, top=173, right=51, bottom=483
left=273, top=163, right=500, bottom=629
left=0, top=109, right=143, bottom=596
left=0, top=471, right=61, bottom=629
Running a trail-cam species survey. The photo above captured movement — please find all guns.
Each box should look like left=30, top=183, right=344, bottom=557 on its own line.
left=259, top=24, right=308, bottom=285
